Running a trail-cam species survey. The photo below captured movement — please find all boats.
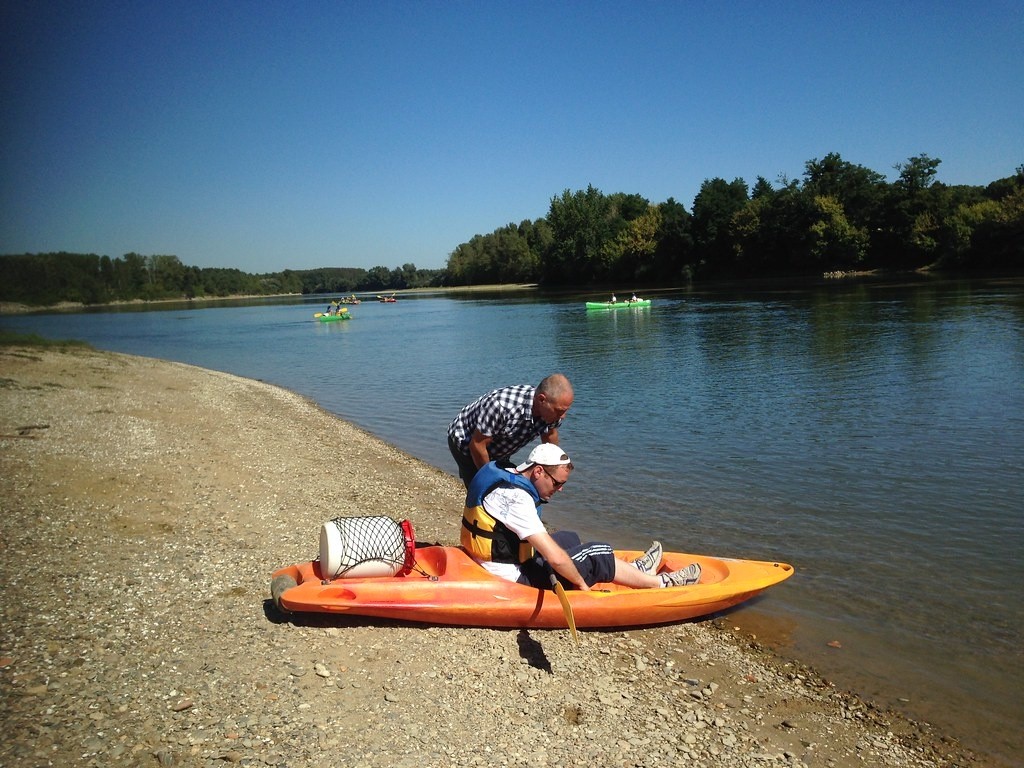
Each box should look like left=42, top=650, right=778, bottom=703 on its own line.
left=585, top=301, right=651, bottom=310
left=271, top=544, right=797, bottom=629
left=380, top=299, right=397, bottom=303
left=319, top=313, right=351, bottom=322
left=342, top=298, right=362, bottom=305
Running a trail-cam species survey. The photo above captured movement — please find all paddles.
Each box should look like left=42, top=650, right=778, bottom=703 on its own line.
left=377, top=295, right=383, bottom=298
left=541, top=557, right=581, bottom=648
left=314, top=308, right=347, bottom=318
left=391, top=293, right=395, bottom=297
left=332, top=301, right=352, bottom=320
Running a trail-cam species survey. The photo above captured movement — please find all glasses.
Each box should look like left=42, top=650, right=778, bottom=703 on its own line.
left=542, top=467, right=566, bottom=489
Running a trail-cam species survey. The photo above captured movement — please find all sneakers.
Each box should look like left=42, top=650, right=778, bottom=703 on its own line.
left=658, top=563, right=702, bottom=588
left=636, top=540, right=662, bottom=578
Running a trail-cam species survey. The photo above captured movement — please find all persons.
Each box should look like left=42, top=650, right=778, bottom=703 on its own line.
left=461, top=443, right=702, bottom=591
left=630, top=293, right=636, bottom=302
left=608, top=293, right=616, bottom=304
left=325, top=306, right=333, bottom=316
left=445, top=375, right=574, bottom=491
left=335, top=305, right=341, bottom=315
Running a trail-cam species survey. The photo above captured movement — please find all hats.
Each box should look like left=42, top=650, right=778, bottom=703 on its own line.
left=516, top=441, right=571, bottom=473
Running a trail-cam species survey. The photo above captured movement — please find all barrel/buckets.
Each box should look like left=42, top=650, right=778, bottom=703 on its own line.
left=320, top=516, right=415, bottom=579
